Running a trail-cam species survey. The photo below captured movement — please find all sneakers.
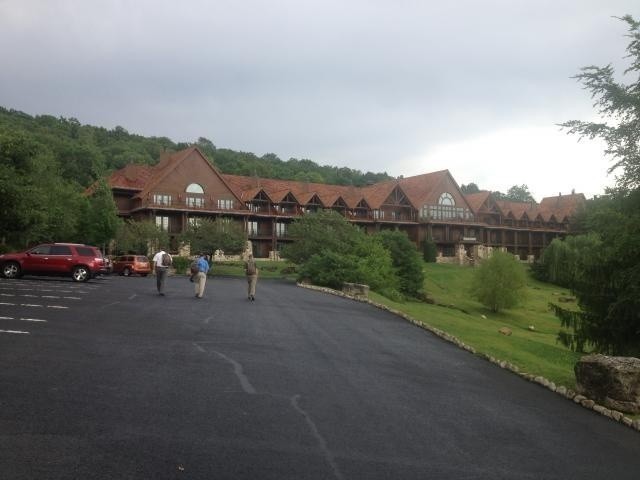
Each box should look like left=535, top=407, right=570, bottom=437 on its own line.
left=199, top=297, right=203, bottom=299
left=251, top=295, right=255, bottom=301
left=195, top=294, right=198, bottom=297
left=157, top=293, right=160, bottom=295
left=160, top=293, right=164, bottom=296
left=249, top=296, right=251, bottom=300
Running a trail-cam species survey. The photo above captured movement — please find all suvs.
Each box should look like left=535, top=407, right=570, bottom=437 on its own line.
left=0, top=242, right=107, bottom=282
left=90, top=254, right=152, bottom=278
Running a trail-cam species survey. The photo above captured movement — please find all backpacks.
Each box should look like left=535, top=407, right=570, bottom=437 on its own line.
left=191, top=261, right=199, bottom=273
left=162, top=254, right=171, bottom=265
left=247, top=262, right=256, bottom=275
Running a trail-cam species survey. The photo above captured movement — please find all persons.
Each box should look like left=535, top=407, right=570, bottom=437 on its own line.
left=244, top=254, right=259, bottom=302
left=152, top=246, right=173, bottom=296
left=194, top=253, right=210, bottom=298
